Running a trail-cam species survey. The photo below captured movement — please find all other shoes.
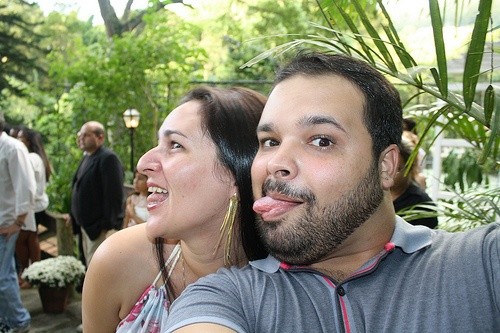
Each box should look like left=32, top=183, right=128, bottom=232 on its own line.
left=0, top=319, right=31, bottom=333
left=17, top=271, right=24, bottom=284
left=21, top=281, right=34, bottom=289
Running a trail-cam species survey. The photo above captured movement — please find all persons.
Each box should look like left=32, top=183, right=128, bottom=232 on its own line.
left=79, top=84, right=270, bottom=332
left=122, top=169, right=152, bottom=227
left=391, top=139, right=441, bottom=231
left=167, top=51, right=500, bottom=333
left=0, top=121, right=39, bottom=332
left=58, top=120, right=127, bottom=333
left=395, top=116, right=428, bottom=191
left=7, top=126, right=52, bottom=288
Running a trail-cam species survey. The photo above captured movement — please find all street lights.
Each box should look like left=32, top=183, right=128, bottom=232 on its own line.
left=122, top=108, right=141, bottom=171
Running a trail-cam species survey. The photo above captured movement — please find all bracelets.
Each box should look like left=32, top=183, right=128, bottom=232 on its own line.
left=14, top=218, right=25, bottom=227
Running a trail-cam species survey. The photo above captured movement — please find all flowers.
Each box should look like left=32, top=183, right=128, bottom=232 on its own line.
left=19, top=255, right=87, bottom=288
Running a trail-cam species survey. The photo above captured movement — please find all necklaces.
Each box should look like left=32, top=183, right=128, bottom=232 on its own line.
left=179, top=251, right=188, bottom=289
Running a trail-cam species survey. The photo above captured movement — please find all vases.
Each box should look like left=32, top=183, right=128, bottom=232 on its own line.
left=39, top=287, right=69, bottom=313
